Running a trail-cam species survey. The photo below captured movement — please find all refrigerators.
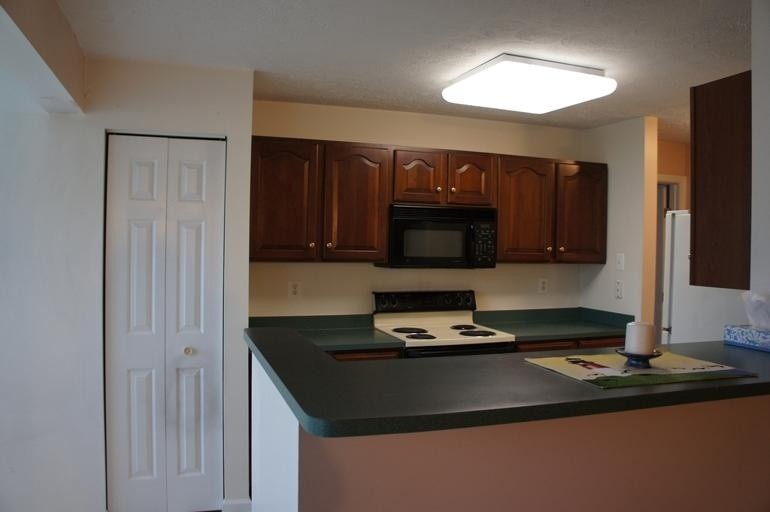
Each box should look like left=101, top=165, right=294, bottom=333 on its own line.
left=660, top=209, right=750, bottom=344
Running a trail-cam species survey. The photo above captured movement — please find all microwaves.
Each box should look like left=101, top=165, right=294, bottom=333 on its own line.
left=388, top=202, right=500, bottom=269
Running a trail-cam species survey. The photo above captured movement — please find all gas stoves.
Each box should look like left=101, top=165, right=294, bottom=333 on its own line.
left=375, top=323, right=516, bottom=346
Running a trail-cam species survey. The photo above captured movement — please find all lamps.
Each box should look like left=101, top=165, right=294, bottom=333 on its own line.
left=442, top=54, right=618, bottom=115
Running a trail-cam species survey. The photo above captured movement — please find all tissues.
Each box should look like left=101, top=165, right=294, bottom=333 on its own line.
left=724, top=290, right=770, bottom=352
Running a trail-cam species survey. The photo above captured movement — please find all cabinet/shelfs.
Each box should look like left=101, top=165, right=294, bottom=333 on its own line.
left=497, top=153, right=608, bottom=264
left=249, top=135, right=392, bottom=264
left=392, top=144, right=497, bottom=207
left=689, top=69, right=753, bottom=291
